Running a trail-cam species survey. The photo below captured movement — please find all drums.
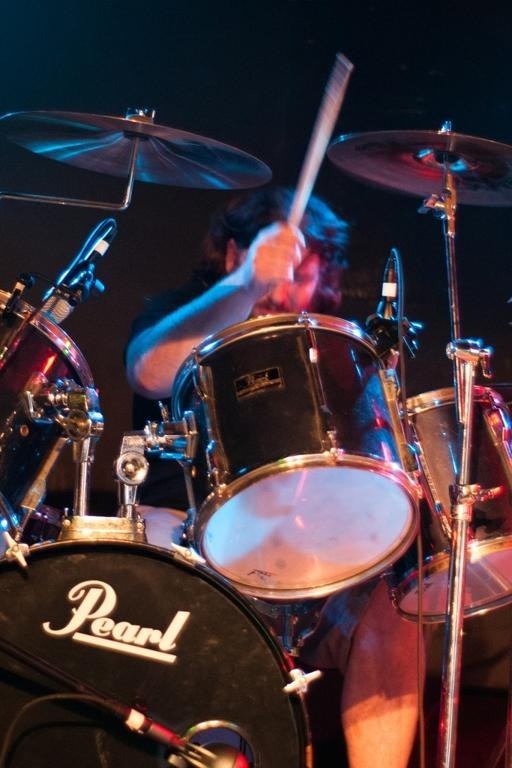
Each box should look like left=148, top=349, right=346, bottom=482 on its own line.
left=0, top=541, right=314, bottom=767
left=172, top=310, right=421, bottom=600
left=389, top=384, right=512, bottom=624
left=0, top=286, right=101, bottom=525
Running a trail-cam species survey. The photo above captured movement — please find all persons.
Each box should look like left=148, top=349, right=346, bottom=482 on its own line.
left=124, top=183, right=427, bottom=768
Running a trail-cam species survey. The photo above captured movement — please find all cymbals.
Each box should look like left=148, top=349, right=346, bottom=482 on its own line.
left=0, top=111, right=274, bottom=190
left=325, top=129, right=512, bottom=205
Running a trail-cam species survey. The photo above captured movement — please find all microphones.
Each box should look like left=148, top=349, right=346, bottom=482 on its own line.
left=36, top=227, right=119, bottom=325
left=364, top=260, right=401, bottom=346
